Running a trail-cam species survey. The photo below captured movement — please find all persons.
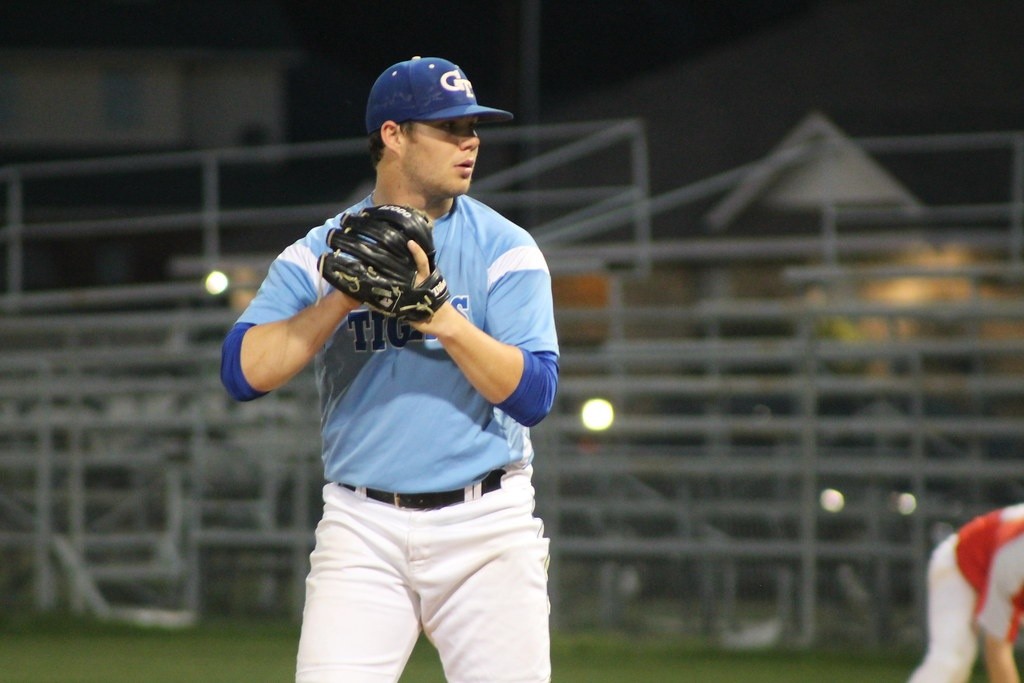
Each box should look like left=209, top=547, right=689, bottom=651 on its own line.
left=219, top=54, right=559, bottom=683
left=906, top=501, right=1024, bottom=683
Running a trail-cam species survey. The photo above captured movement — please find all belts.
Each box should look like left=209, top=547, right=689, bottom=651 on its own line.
left=338, top=469, right=507, bottom=509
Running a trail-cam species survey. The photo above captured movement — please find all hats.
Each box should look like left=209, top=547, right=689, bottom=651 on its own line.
left=365, top=55, right=514, bottom=135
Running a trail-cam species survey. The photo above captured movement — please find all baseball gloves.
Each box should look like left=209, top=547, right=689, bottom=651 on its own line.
left=315, top=203, right=452, bottom=323
